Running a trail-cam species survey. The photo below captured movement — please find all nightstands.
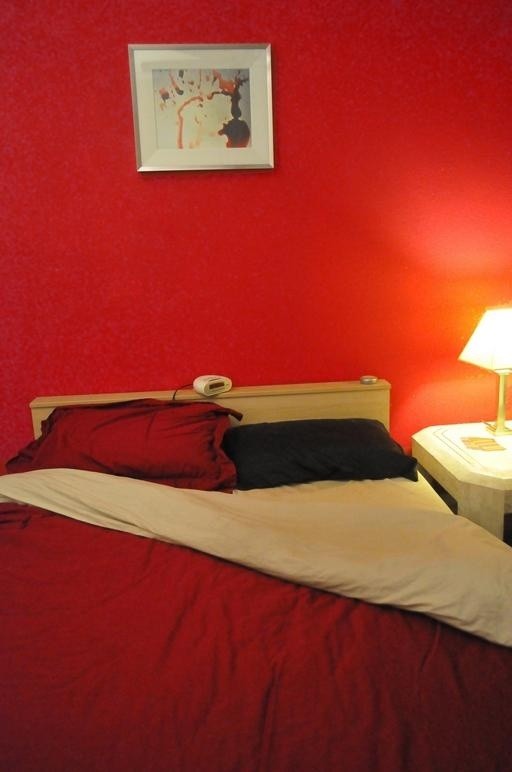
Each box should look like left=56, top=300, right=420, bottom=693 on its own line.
left=411, top=420, right=512, bottom=541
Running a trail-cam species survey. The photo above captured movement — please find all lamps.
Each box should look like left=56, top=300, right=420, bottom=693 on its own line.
left=457, top=309, right=512, bottom=436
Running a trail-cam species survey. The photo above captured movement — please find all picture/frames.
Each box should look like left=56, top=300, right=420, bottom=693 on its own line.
left=128, top=43, right=274, bottom=173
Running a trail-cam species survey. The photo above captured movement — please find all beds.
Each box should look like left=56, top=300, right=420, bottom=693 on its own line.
left=1, top=378, right=510, bottom=770
